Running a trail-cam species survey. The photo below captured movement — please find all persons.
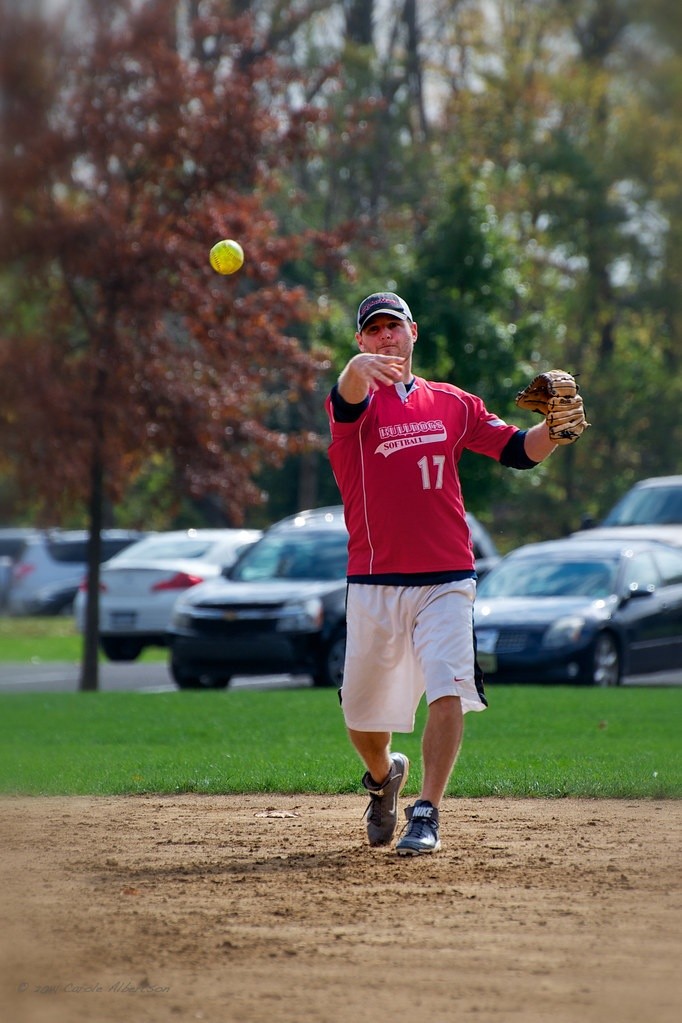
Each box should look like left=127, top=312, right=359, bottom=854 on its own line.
left=326, top=293, right=586, bottom=858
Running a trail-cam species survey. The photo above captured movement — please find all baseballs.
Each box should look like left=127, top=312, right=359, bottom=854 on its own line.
left=209, top=239, right=244, bottom=275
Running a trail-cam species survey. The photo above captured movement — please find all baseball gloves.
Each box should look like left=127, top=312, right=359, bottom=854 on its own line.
left=515, top=367, right=594, bottom=445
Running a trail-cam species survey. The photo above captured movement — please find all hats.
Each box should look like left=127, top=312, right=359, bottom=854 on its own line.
left=356, top=292, right=413, bottom=331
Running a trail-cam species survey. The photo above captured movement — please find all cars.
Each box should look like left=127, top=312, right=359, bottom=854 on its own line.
left=575, top=475, right=682, bottom=546
left=0, top=527, right=146, bottom=618
left=74, top=527, right=267, bottom=666
left=167, top=502, right=500, bottom=694
left=479, top=540, right=682, bottom=690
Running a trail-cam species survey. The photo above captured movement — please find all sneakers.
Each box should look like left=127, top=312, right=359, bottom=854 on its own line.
left=361, top=751, right=410, bottom=848
left=395, top=800, right=441, bottom=855
